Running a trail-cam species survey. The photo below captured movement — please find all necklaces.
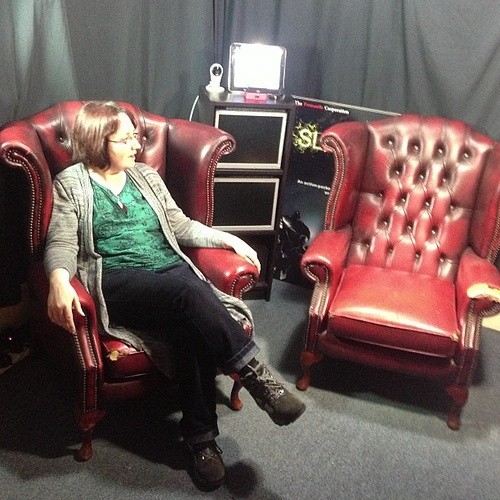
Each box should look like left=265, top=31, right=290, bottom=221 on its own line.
left=115, top=195, right=124, bottom=210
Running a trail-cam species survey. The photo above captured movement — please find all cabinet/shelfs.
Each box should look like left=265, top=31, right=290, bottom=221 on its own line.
left=197, top=86, right=295, bottom=302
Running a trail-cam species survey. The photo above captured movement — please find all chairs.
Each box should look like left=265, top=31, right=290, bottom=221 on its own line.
left=291, top=113, right=500, bottom=431
left=0, top=99, right=259, bottom=462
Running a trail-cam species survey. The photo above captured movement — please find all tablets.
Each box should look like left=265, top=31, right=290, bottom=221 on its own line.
left=228, top=42, right=287, bottom=95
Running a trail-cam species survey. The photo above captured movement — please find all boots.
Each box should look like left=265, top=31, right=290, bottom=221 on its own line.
left=239, top=361, right=306, bottom=426
left=182, top=434, right=228, bottom=488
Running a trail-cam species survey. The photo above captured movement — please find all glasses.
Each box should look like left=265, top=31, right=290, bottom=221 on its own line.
left=107, top=130, right=138, bottom=143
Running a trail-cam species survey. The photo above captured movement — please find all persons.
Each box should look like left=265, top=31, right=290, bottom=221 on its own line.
left=42, top=100, right=307, bottom=486
